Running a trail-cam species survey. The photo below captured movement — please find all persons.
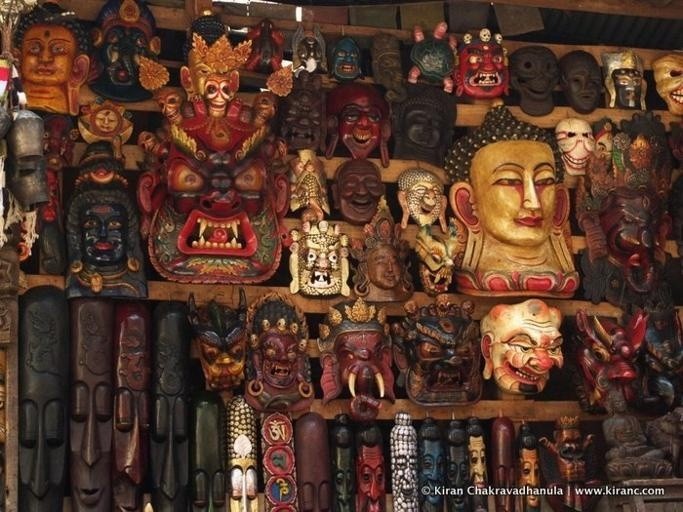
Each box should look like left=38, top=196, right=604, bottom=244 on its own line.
left=0, top=0, right=683, bottom=512
